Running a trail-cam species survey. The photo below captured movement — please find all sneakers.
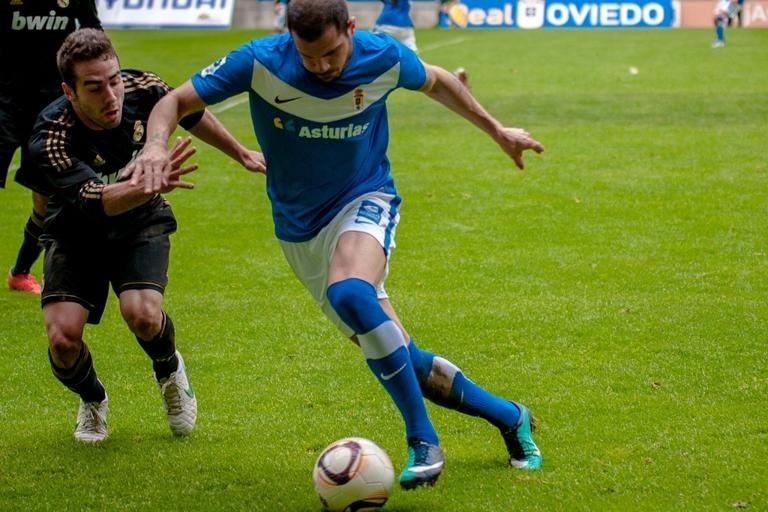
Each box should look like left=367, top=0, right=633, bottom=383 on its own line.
left=455, top=66, right=468, bottom=90
left=7, top=267, right=41, bottom=294
left=398, top=440, right=444, bottom=488
left=75, top=378, right=108, bottom=442
left=503, top=400, right=543, bottom=471
left=153, top=351, right=197, bottom=435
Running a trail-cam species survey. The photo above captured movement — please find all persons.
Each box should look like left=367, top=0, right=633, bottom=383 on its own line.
left=373, top=0, right=470, bottom=101
left=120, top=0, right=544, bottom=490
left=29, top=27, right=269, bottom=445
left=0, top=0, right=103, bottom=296
left=711, top=0, right=744, bottom=48
left=271, top=0, right=293, bottom=35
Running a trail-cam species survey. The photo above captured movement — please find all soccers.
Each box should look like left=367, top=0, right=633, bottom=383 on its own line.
left=313, top=436, right=395, bottom=512
